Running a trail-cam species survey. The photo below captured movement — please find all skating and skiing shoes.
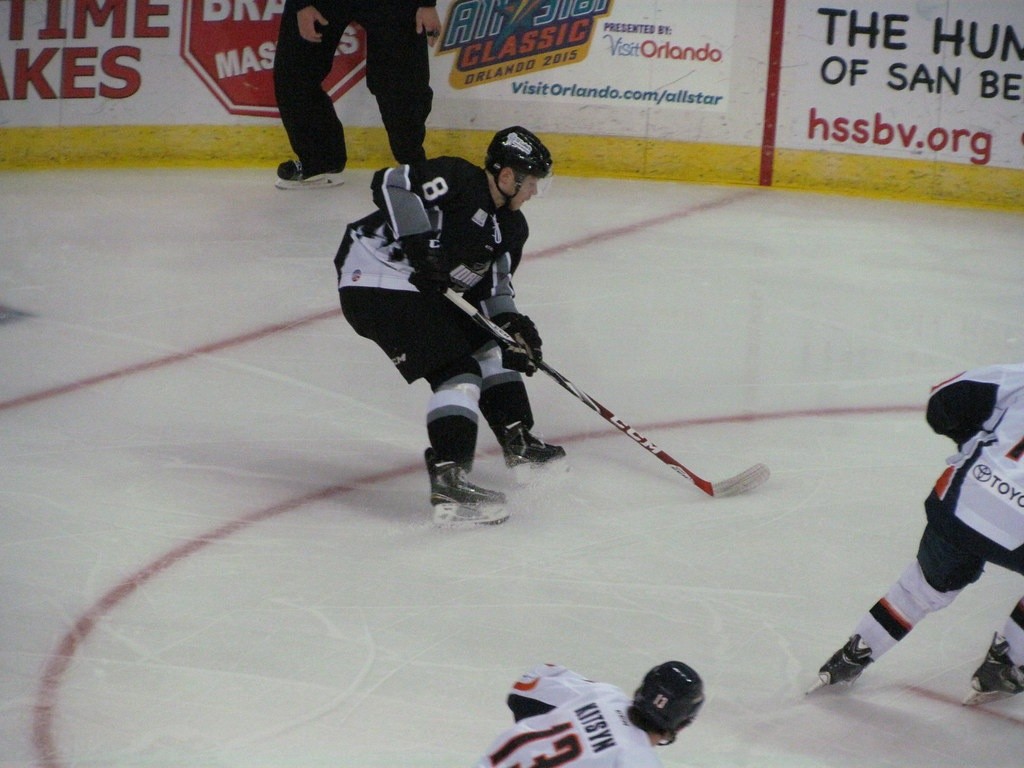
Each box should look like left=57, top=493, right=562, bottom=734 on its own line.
left=963, top=630, right=1024, bottom=706
left=806, top=635, right=875, bottom=694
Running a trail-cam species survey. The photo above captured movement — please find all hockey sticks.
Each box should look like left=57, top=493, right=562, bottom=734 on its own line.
left=412, top=271, right=771, bottom=500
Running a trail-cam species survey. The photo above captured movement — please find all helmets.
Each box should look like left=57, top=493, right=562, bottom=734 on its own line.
left=485, top=126, right=553, bottom=179
left=633, top=660, right=706, bottom=733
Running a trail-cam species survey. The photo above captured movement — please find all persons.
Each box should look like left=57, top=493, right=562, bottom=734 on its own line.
left=478, top=659, right=706, bottom=768
left=332, top=124, right=567, bottom=527
left=804, top=361, right=1023, bottom=710
left=272, top=0, right=442, bottom=191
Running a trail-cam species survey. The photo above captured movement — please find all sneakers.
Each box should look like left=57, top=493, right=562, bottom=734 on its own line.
left=276, top=159, right=346, bottom=189
left=498, top=421, right=569, bottom=485
left=424, top=447, right=510, bottom=525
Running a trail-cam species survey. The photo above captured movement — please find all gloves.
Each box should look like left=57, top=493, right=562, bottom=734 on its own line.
left=493, top=316, right=542, bottom=376
left=408, top=239, right=455, bottom=309
